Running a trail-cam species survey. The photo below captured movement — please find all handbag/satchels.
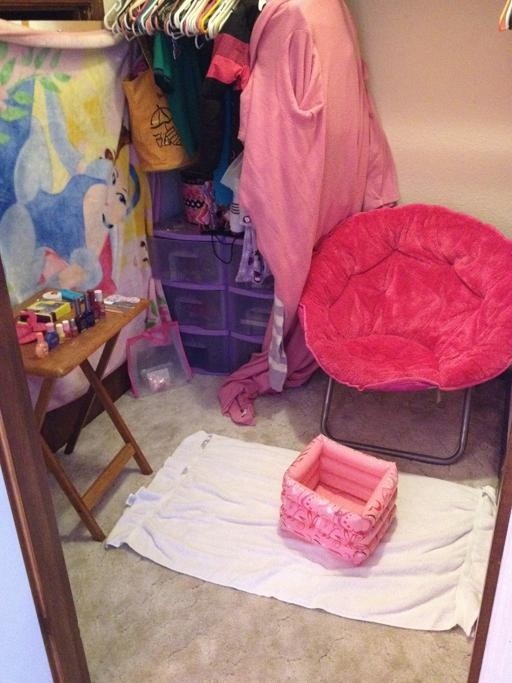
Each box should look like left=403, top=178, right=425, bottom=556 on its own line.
left=123, top=66, right=197, bottom=176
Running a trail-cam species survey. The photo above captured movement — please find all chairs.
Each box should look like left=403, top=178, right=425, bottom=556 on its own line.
left=296, top=205, right=510, bottom=466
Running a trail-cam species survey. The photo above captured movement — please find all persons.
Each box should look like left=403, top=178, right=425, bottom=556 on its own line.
left=27, top=139, right=153, bottom=300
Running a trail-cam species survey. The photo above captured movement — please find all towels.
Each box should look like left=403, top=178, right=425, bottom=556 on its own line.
left=101, top=430, right=495, bottom=636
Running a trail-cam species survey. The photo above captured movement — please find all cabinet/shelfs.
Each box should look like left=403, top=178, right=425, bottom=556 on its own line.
left=146, top=217, right=289, bottom=378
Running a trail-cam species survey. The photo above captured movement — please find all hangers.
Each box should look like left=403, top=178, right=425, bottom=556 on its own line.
left=102, top=0, right=266, bottom=52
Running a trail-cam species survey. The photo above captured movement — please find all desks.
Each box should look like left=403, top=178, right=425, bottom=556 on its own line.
left=9, top=286, right=154, bottom=543
